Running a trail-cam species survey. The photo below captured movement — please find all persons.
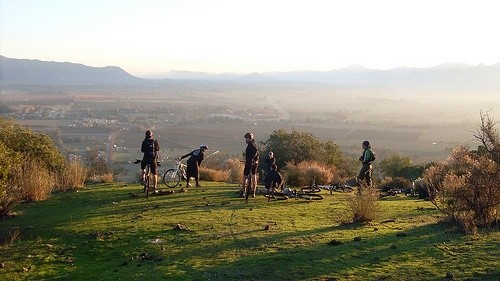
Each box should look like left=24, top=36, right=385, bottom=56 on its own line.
left=241, top=133, right=259, bottom=197
left=355, top=140, right=376, bottom=189
left=140, top=130, right=161, bottom=195
left=177, top=144, right=208, bottom=188
left=263, top=151, right=284, bottom=193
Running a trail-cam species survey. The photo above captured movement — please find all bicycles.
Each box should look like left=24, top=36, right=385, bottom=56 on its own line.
left=163, top=157, right=200, bottom=188
left=263, top=174, right=354, bottom=202
left=134, top=159, right=161, bottom=198
left=240, top=160, right=259, bottom=204
left=380, top=178, right=419, bottom=197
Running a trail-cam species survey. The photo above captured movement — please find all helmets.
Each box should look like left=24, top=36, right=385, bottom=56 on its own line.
left=145, top=129, right=153, bottom=136
left=200, top=143, right=208, bottom=149
left=244, top=132, right=254, bottom=142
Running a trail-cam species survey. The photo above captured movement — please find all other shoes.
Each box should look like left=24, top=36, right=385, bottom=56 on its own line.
left=248, top=192, right=255, bottom=197
left=239, top=191, right=245, bottom=198
